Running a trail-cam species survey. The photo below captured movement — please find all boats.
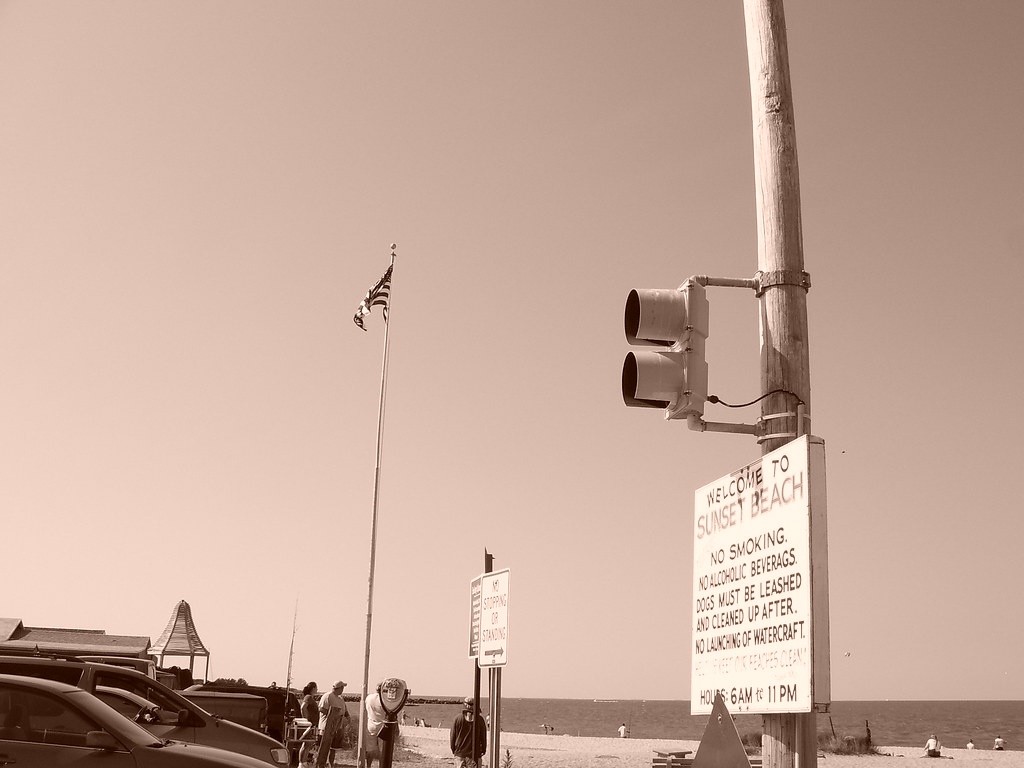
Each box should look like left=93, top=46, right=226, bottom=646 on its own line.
left=595, top=699, right=618, bottom=703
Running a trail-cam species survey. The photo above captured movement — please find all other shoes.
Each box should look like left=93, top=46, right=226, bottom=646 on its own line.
left=298, top=763, right=309, bottom=768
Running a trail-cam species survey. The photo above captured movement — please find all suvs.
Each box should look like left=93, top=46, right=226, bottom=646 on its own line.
left=0, top=655, right=301, bottom=768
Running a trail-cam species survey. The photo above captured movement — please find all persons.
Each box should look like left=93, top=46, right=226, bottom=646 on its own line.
left=924, top=734, right=942, bottom=757
left=618, top=723, right=630, bottom=738
left=486, top=713, right=490, bottom=730
left=967, top=739, right=975, bottom=749
left=450, top=697, right=487, bottom=768
left=993, top=735, right=1008, bottom=750
left=364, top=682, right=389, bottom=768
left=413, top=717, right=431, bottom=727
left=316, top=681, right=350, bottom=768
left=298, top=682, right=320, bottom=768
left=402, top=710, right=409, bottom=726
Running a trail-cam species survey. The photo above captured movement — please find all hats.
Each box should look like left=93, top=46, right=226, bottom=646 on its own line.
left=333, top=681, right=347, bottom=688
left=464, top=697, right=474, bottom=708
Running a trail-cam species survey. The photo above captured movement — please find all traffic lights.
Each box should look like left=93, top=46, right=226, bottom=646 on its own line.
left=622, top=277, right=709, bottom=422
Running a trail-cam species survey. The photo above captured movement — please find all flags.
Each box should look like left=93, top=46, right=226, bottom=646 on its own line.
left=353, top=264, right=393, bottom=330
